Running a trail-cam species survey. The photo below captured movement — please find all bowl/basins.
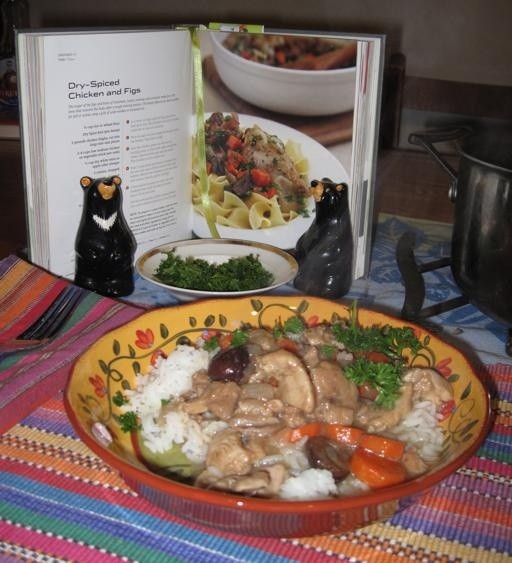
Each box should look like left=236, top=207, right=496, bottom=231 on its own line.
left=62, top=294, right=495, bottom=542
left=206, top=31, right=357, bottom=116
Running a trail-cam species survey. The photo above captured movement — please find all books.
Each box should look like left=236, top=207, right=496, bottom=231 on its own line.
left=9, top=18, right=393, bottom=299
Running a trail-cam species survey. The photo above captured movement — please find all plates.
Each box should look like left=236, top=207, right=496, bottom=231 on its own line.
left=183, top=111, right=349, bottom=247
left=0, top=57, right=20, bottom=139
left=136, top=237, right=299, bottom=296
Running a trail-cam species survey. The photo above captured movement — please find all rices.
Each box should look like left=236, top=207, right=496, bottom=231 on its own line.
left=94, top=342, right=445, bottom=501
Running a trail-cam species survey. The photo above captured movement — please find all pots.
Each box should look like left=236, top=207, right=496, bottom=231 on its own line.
left=398, top=114, right=511, bottom=325
